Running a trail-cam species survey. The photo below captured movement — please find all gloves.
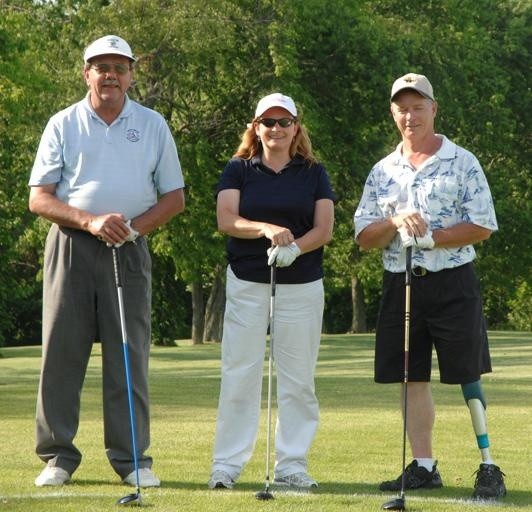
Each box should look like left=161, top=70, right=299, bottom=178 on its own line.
left=266, top=241, right=302, bottom=268
left=97, top=219, right=139, bottom=248
left=397, top=226, right=435, bottom=249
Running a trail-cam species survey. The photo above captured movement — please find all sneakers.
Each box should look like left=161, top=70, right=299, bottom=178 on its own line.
left=35, top=466, right=70, bottom=487
left=378, top=459, right=443, bottom=491
left=272, top=472, right=318, bottom=488
left=208, top=470, right=233, bottom=489
left=467, top=464, right=506, bottom=499
left=123, top=467, right=161, bottom=488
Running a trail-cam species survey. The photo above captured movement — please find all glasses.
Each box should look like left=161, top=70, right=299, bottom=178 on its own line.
left=90, top=64, right=128, bottom=75
left=257, top=118, right=296, bottom=127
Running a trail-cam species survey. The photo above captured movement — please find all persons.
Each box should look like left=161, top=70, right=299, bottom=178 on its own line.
left=27, top=34, right=186, bottom=488
left=206, top=91, right=336, bottom=489
left=353, top=72, right=508, bottom=502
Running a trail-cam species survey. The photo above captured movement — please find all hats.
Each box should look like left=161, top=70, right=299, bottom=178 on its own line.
left=83, top=35, right=135, bottom=64
left=255, top=93, right=297, bottom=117
left=391, top=73, right=435, bottom=102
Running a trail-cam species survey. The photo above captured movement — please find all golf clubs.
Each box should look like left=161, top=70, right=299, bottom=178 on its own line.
left=384, top=246, right=411, bottom=511
left=113, top=244, right=144, bottom=506
left=257, top=242, right=275, bottom=503
